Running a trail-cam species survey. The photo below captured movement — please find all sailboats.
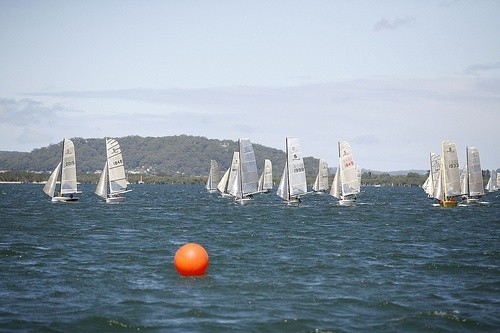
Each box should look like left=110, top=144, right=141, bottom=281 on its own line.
left=93, top=138, right=133, bottom=205
left=331, top=140, right=362, bottom=208
left=435, top=142, right=463, bottom=208
left=231, top=139, right=260, bottom=205
left=42, top=138, right=79, bottom=204
left=312, top=158, right=330, bottom=196
left=484, top=170, right=500, bottom=193
left=205, top=159, right=219, bottom=193
left=422, top=152, right=443, bottom=199
left=276, top=136, right=307, bottom=205
left=138, top=178, right=145, bottom=185
left=258, top=159, right=273, bottom=195
left=458, top=146, right=492, bottom=208
left=217, top=151, right=239, bottom=198
